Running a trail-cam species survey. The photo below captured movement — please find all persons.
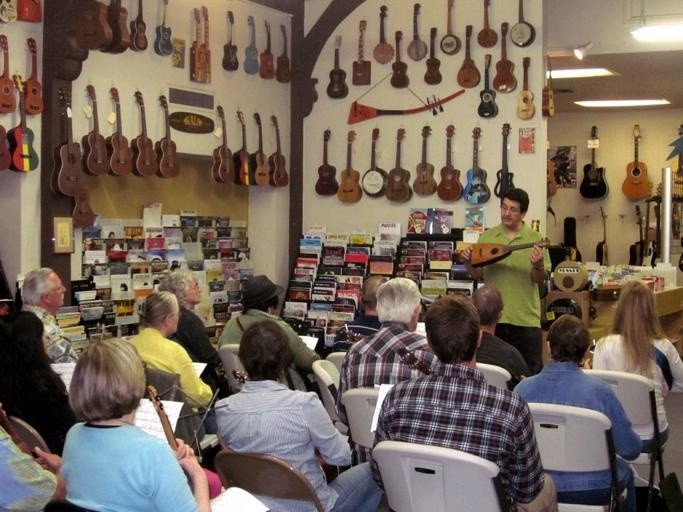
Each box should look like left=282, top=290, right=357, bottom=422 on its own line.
left=369, top=294, right=558, bottom=512
left=209, top=321, right=386, bottom=512
left=217, top=273, right=321, bottom=399
left=121, top=290, right=223, bottom=455
left=1, top=311, right=75, bottom=457
left=591, top=280, right=683, bottom=454
left=336, top=278, right=436, bottom=471
left=1, top=422, right=70, bottom=511
left=512, top=314, right=643, bottom=512
left=461, top=188, right=552, bottom=374
left=59, top=339, right=222, bottom=512
left=19, top=268, right=79, bottom=362
left=159, top=271, right=231, bottom=397
left=331, top=275, right=389, bottom=352
left=469, top=284, right=532, bottom=390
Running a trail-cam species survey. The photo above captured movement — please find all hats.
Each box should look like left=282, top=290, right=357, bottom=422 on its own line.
left=240, top=275, right=284, bottom=308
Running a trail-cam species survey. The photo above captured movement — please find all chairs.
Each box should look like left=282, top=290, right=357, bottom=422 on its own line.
left=9, top=416, right=50, bottom=457
left=325, top=351, right=348, bottom=376
left=213, top=451, right=324, bottom=511
left=340, top=387, right=385, bottom=450
left=219, top=344, right=250, bottom=393
left=312, top=360, right=346, bottom=423
left=371, top=441, right=504, bottom=511
left=476, top=360, right=513, bottom=390
left=582, top=368, right=666, bottom=511
left=528, top=401, right=624, bottom=512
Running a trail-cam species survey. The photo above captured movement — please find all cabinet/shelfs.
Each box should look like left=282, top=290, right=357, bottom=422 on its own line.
left=54, top=215, right=253, bottom=357
left=278, top=225, right=484, bottom=354
left=589, top=286, right=683, bottom=360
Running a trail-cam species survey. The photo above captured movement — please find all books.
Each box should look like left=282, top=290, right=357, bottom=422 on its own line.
left=60, top=325, right=89, bottom=350
left=209, top=277, right=244, bottom=323
left=280, top=208, right=472, bottom=285
left=336, top=286, right=361, bottom=320
left=72, top=277, right=118, bottom=339
left=111, top=277, right=159, bottom=337
left=55, top=306, right=86, bottom=327
left=283, top=285, right=335, bottom=347
left=82, top=208, right=250, bottom=277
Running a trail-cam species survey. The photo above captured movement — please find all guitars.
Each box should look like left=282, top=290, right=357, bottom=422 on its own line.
left=397, top=347, right=430, bottom=375
left=423, top=27, right=442, bottom=85
left=435, top=215, right=449, bottom=234
left=386, top=128, right=412, bottom=203
left=0, top=126, right=12, bottom=170
left=244, top=16, right=259, bottom=74
left=260, top=20, right=276, bottom=80
left=132, top=92, right=157, bottom=177
left=211, top=104, right=235, bottom=185
left=232, top=369, right=248, bottom=384
left=315, top=129, right=339, bottom=196
left=517, top=56, right=535, bottom=119
left=148, top=386, right=179, bottom=458
left=493, top=123, right=516, bottom=198
left=7, top=75, right=38, bottom=171
left=233, top=111, right=254, bottom=186
left=23, top=38, right=43, bottom=115
left=436, top=125, right=464, bottom=201
left=52, top=87, right=85, bottom=197
left=547, top=140, right=557, bottom=197
left=0, top=408, right=33, bottom=460
left=72, top=187, right=93, bottom=227
left=337, top=130, right=362, bottom=204
left=622, top=124, right=649, bottom=200
left=493, top=22, right=517, bottom=94
left=477, top=54, right=499, bottom=118
left=82, top=85, right=110, bottom=175
left=155, top=95, right=179, bottom=177
left=327, top=36, right=349, bottom=100
left=579, top=125, right=609, bottom=201
left=222, top=11, right=239, bottom=71
left=391, top=32, right=409, bottom=89
left=267, top=116, right=288, bottom=187
left=470, top=238, right=558, bottom=268
left=0, top=35, right=17, bottom=114
left=130, top=0, right=148, bottom=52
left=276, top=25, right=290, bottom=83
left=463, top=128, right=491, bottom=205
left=629, top=205, right=644, bottom=266
left=362, top=127, right=383, bottom=201
left=106, top=87, right=132, bottom=175
left=154, top=0, right=173, bottom=56
left=408, top=216, right=421, bottom=234
left=413, top=125, right=437, bottom=194
left=251, top=113, right=269, bottom=186
left=335, top=323, right=365, bottom=344
left=542, top=54, right=555, bottom=119
left=101, top=0, right=130, bottom=54
left=596, top=206, right=608, bottom=266
left=641, top=197, right=657, bottom=268
left=407, top=2, right=430, bottom=64
